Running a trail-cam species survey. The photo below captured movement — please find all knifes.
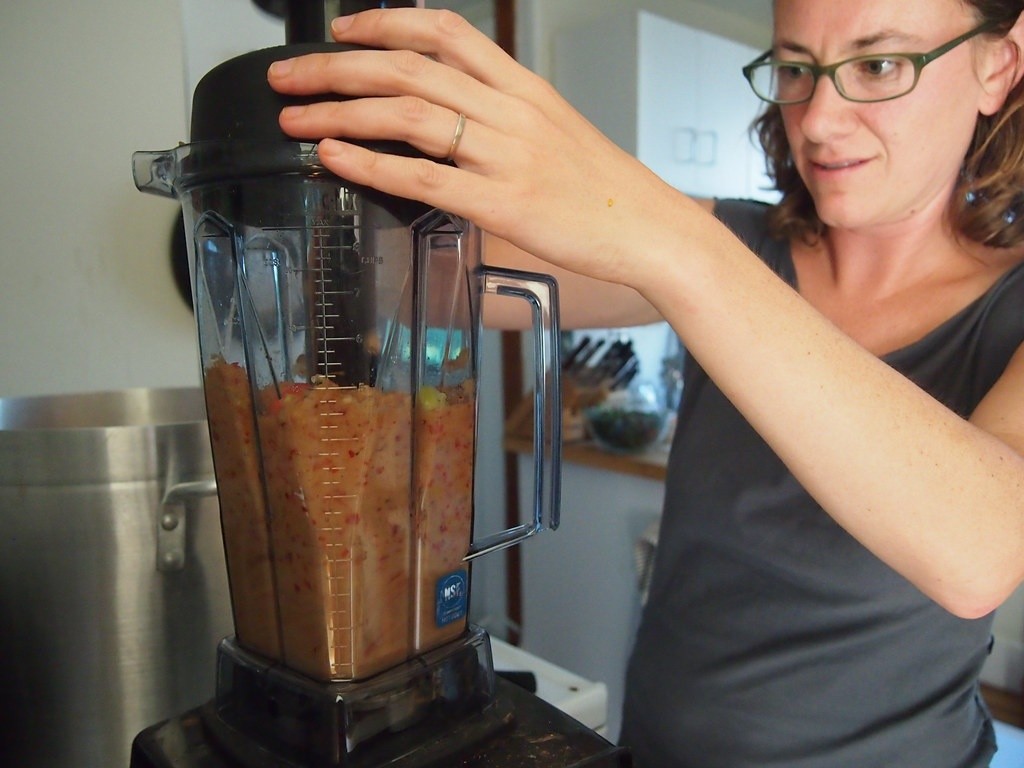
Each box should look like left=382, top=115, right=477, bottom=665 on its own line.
left=558, top=332, right=639, bottom=392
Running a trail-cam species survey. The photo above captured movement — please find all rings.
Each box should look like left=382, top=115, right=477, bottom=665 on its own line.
left=448, top=111, right=467, bottom=161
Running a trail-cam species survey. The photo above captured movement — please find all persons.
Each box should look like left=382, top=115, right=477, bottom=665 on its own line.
left=268, top=0, right=1024, bottom=768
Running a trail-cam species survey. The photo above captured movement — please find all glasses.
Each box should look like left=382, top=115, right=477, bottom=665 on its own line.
left=741, top=14, right=1004, bottom=104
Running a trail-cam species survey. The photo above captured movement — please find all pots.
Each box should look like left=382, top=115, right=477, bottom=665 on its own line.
left=0, top=385, right=234, bottom=768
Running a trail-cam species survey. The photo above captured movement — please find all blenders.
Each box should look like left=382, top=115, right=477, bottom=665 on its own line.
left=131, top=1, right=629, bottom=768
